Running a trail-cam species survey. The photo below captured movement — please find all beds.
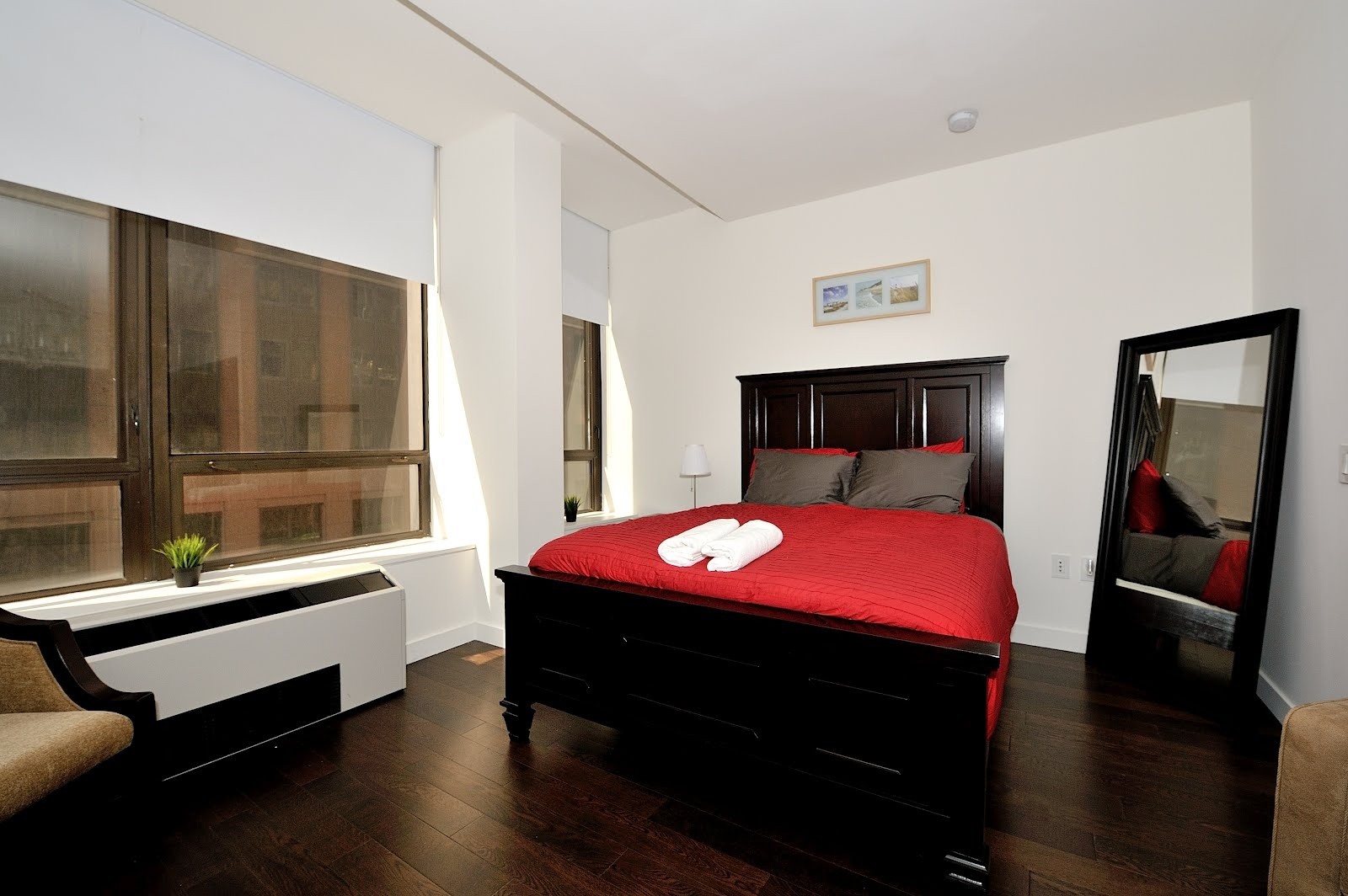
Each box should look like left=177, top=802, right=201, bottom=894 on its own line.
left=1111, top=368, right=1251, bottom=689
left=496, top=356, right=1018, bottom=896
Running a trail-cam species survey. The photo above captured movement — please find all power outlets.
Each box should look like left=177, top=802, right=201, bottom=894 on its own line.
left=1081, top=558, right=1097, bottom=581
left=1051, top=553, right=1069, bottom=579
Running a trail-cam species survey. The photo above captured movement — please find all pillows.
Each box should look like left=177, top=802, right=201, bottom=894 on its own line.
left=1130, top=463, right=1229, bottom=539
left=737, top=437, right=976, bottom=515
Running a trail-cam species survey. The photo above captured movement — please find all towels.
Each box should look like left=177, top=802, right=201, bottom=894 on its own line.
left=656, top=517, right=784, bottom=574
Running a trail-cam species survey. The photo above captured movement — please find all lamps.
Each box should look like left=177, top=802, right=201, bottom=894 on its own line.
left=679, top=443, right=710, bottom=509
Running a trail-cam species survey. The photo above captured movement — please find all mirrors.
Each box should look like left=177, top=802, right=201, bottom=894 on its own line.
left=1082, top=307, right=1300, bottom=720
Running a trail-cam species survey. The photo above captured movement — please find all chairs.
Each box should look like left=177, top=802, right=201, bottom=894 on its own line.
left=0, top=607, right=158, bottom=896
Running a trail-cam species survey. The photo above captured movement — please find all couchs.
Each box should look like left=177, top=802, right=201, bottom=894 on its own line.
left=1266, top=699, right=1347, bottom=896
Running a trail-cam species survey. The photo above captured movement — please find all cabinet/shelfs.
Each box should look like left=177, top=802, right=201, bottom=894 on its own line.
left=70, top=564, right=408, bottom=791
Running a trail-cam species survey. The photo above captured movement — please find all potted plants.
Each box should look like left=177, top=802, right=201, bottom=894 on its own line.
left=152, top=533, right=220, bottom=587
left=565, top=495, right=583, bottom=522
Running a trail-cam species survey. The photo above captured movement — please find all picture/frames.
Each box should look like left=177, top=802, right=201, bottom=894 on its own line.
left=1146, top=353, right=1157, bottom=371
left=812, top=257, right=931, bottom=327
